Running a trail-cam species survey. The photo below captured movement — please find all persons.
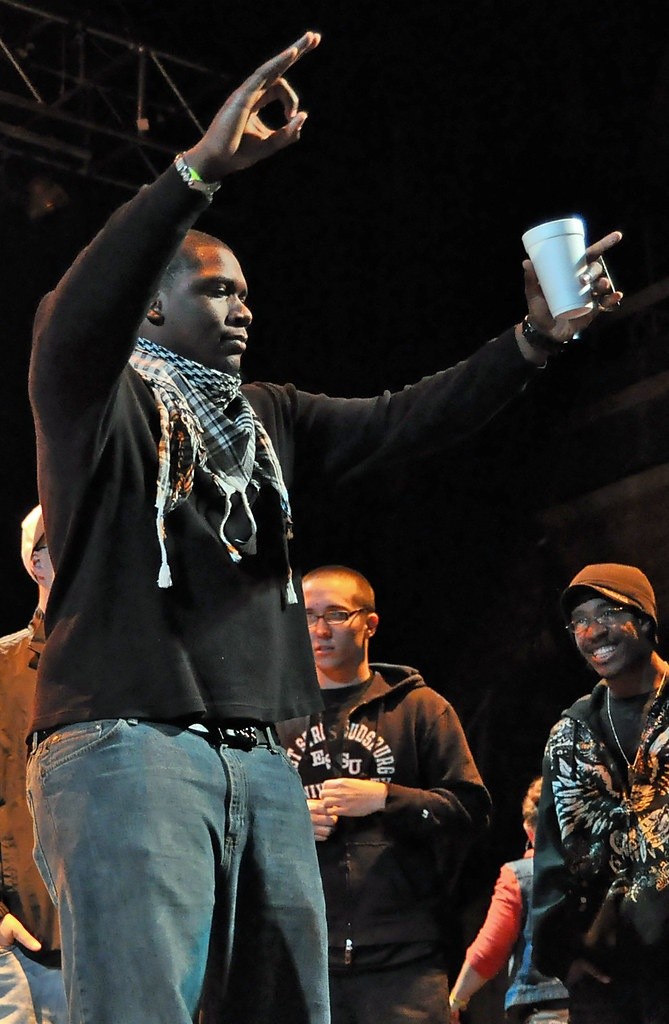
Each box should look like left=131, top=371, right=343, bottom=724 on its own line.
left=0, top=505, right=66, bottom=1024
left=532, top=562, right=669, bottom=1024
left=24, top=32, right=623, bottom=1024
left=301, top=568, right=490, bottom=1024
left=450, top=776, right=569, bottom=1024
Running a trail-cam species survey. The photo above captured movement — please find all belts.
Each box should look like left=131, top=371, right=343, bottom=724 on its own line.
left=25, top=718, right=280, bottom=748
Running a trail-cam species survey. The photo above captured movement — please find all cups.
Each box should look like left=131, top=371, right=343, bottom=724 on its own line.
left=522, top=218, right=589, bottom=319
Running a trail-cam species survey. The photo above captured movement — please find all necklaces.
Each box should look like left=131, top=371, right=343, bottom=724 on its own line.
left=605, top=663, right=666, bottom=784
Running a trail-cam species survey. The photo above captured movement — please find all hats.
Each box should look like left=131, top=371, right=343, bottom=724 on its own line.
left=21, top=503, right=49, bottom=584
left=561, top=563, right=658, bottom=623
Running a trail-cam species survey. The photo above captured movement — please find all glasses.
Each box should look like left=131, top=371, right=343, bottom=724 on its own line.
left=304, top=607, right=364, bottom=627
left=565, top=607, right=629, bottom=633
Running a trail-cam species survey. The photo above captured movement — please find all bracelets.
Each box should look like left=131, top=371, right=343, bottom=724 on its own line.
left=175, top=152, right=221, bottom=204
left=521, top=315, right=573, bottom=358
left=450, top=989, right=470, bottom=1006
